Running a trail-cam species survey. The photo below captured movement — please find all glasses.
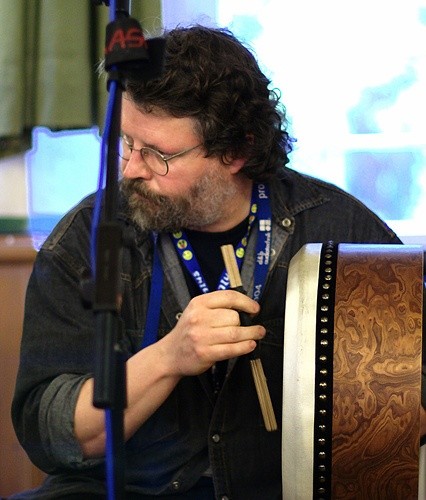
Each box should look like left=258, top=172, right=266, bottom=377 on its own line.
left=118, top=138, right=209, bottom=176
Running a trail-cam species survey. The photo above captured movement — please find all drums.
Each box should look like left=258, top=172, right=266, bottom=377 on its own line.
left=266, top=241, right=425, bottom=500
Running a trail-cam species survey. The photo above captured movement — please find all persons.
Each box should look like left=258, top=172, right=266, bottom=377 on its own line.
left=2, top=21, right=407, bottom=499
